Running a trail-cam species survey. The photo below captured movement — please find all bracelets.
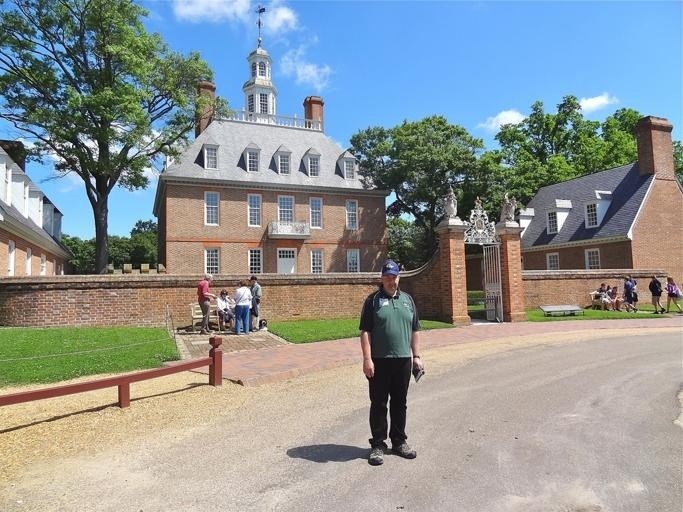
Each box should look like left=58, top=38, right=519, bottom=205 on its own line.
left=413, top=356, right=420, bottom=359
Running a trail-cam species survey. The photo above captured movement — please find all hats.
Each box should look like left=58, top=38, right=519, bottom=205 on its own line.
left=249, top=276, right=257, bottom=281
left=381, top=259, right=400, bottom=277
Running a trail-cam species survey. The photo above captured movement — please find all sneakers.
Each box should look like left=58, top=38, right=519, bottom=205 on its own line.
left=234, top=327, right=260, bottom=335
left=607, top=306, right=640, bottom=313
left=651, top=308, right=683, bottom=314
left=368, top=444, right=385, bottom=466
left=200, top=330, right=212, bottom=335
left=391, top=440, right=417, bottom=459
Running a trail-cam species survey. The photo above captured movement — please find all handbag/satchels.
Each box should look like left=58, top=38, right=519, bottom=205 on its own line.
left=655, top=287, right=663, bottom=295
left=231, top=303, right=237, bottom=313
left=208, top=296, right=216, bottom=305
left=252, top=298, right=261, bottom=305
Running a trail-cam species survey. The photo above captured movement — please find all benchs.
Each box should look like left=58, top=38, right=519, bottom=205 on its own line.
left=589, top=290, right=621, bottom=310
left=188, top=302, right=260, bottom=332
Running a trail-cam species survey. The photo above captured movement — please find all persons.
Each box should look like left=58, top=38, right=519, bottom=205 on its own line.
left=359, top=260, right=422, bottom=466
left=234, top=280, right=252, bottom=335
left=596, top=276, right=638, bottom=312
left=665, top=277, right=683, bottom=313
left=649, top=274, right=665, bottom=314
left=216, top=289, right=234, bottom=331
left=197, top=274, right=217, bottom=335
left=248, top=276, right=262, bottom=332
